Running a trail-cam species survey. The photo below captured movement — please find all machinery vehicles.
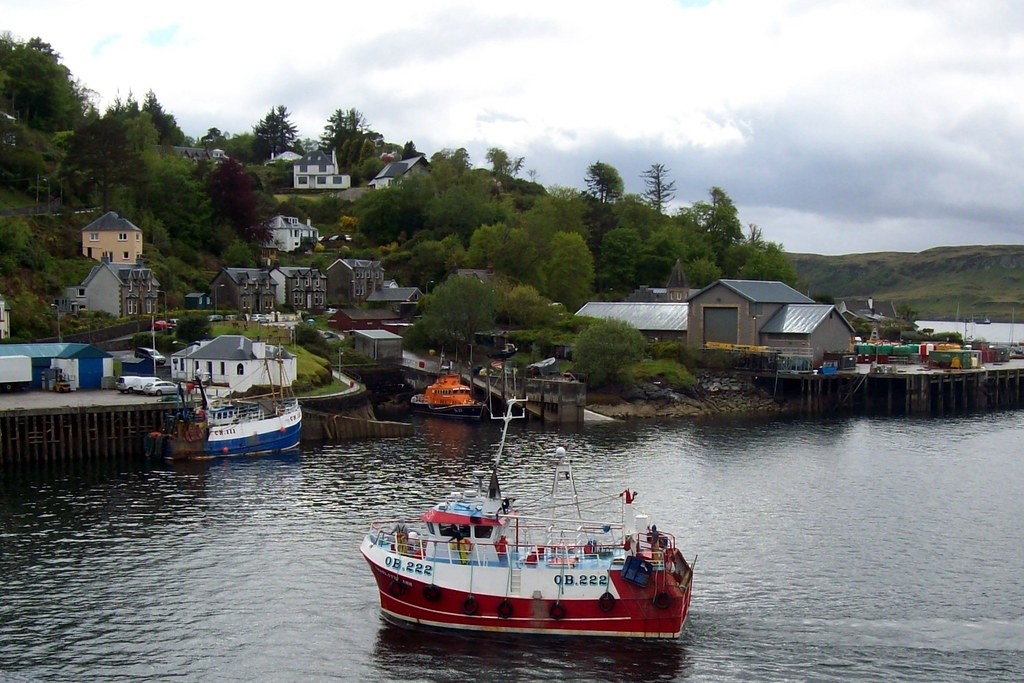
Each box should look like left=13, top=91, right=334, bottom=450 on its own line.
left=52, top=369, right=71, bottom=392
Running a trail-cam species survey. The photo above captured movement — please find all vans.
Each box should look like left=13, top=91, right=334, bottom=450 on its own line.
left=132, top=377, right=163, bottom=393
left=143, top=381, right=182, bottom=396
left=114, top=376, right=143, bottom=393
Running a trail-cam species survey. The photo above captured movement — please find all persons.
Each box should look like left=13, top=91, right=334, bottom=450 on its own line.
left=441, top=524, right=456, bottom=536
left=449, top=526, right=469, bottom=564
left=390, top=518, right=408, bottom=556
left=648, top=525, right=659, bottom=559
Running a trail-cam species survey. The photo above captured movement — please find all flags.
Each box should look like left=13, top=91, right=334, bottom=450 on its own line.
left=625, top=490, right=638, bottom=504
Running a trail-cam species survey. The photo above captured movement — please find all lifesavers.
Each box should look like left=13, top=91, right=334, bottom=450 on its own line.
left=548, top=602, right=567, bottom=620
left=388, top=579, right=405, bottom=596
left=598, top=592, right=616, bottom=612
left=424, top=583, right=440, bottom=602
left=654, top=591, right=673, bottom=609
left=462, top=596, right=478, bottom=615
left=457, top=539, right=472, bottom=554
left=497, top=601, right=512, bottom=619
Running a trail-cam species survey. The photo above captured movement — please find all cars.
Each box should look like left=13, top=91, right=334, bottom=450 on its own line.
left=325, top=309, right=335, bottom=313
left=170, top=319, right=183, bottom=325
left=207, top=315, right=224, bottom=322
left=250, top=314, right=269, bottom=324
left=153, top=321, right=173, bottom=331
left=225, top=315, right=237, bottom=320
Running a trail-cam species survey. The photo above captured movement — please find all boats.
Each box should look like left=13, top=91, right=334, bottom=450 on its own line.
left=359, top=469, right=693, bottom=642
left=155, top=396, right=303, bottom=460
left=410, top=373, right=488, bottom=421
left=525, top=357, right=557, bottom=375
left=487, top=348, right=518, bottom=358
